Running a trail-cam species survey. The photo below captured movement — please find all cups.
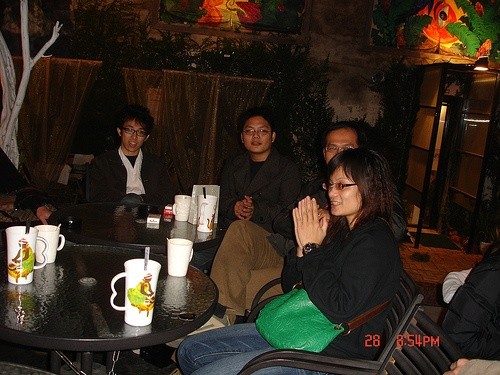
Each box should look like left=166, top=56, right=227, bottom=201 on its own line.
left=166, top=237, right=194, bottom=278
left=109, top=258, right=162, bottom=328
left=195, top=195, right=217, bottom=233
left=33, top=224, right=66, bottom=264
left=5, top=225, right=49, bottom=285
left=173, top=194, right=191, bottom=222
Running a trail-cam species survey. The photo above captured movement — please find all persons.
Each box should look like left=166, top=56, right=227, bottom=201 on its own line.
left=440, top=238, right=500, bottom=375
left=178, top=146, right=413, bottom=375
left=164, top=120, right=372, bottom=375
left=0, top=147, right=56, bottom=260
left=188, top=107, right=302, bottom=275
left=77, top=105, right=174, bottom=212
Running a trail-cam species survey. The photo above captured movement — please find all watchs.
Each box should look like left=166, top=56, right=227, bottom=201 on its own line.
left=302, top=242, right=320, bottom=255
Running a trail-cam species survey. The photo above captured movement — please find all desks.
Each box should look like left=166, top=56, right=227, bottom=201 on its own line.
left=0, top=244, right=219, bottom=375
left=49, top=202, right=219, bottom=253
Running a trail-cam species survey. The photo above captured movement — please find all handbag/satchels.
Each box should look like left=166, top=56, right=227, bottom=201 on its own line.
left=254, top=283, right=347, bottom=354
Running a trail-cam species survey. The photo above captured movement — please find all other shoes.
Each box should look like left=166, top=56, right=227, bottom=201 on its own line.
left=164, top=315, right=230, bottom=348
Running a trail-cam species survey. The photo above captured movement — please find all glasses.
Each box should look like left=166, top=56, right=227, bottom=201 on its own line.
left=321, top=181, right=357, bottom=190
left=324, top=145, right=352, bottom=153
left=120, top=127, right=145, bottom=138
left=242, top=128, right=272, bottom=135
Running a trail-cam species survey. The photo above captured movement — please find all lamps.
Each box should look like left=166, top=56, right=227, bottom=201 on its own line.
left=472, top=58, right=489, bottom=72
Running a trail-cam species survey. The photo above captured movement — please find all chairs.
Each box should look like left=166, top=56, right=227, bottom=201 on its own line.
left=237, top=266, right=468, bottom=375
